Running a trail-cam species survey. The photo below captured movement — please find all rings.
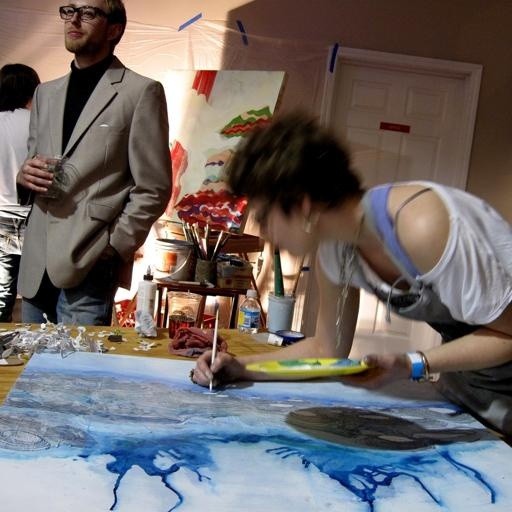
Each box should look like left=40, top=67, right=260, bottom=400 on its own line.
left=188, top=369, right=196, bottom=383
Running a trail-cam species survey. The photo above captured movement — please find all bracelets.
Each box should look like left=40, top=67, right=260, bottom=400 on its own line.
left=406, top=352, right=425, bottom=382
left=417, top=349, right=430, bottom=382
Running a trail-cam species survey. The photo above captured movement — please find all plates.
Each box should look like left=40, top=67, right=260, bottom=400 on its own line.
left=243, top=357, right=374, bottom=382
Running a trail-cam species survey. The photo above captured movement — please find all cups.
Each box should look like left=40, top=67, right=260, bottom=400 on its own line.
left=266, top=292, right=295, bottom=333
left=167, top=291, right=203, bottom=340
left=194, top=258, right=216, bottom=287
left=37, top=154, right=64, bottom=199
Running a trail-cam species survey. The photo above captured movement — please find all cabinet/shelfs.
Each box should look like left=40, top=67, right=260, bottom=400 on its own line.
left=153, top=232, right=265, bottom=329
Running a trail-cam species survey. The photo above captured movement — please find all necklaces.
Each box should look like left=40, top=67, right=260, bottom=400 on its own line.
left=333, top=212, right=368, bottom=352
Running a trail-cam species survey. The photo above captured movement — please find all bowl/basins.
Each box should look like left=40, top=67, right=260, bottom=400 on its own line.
left=276, top=330, right=305, bottom=346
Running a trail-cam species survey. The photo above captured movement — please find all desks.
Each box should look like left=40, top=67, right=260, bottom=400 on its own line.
left=1, top=323, right=349, bottom=406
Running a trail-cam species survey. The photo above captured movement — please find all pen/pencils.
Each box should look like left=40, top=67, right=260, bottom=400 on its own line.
left=181, top=221, right=232, bottom=261
left=205, top=305, right=221, bottom=395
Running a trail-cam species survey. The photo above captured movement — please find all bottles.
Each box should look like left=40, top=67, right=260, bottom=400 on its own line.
left=135, top=265, right=157, bottom=324
left=154, top=239, right=194, bottom=282
left=238, top=289, right=261, bottom=333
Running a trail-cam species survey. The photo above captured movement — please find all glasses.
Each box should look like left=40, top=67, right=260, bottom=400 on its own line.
left=253, top=199, right=281, bottom=229
left=59, top=5, right=118, bottom=23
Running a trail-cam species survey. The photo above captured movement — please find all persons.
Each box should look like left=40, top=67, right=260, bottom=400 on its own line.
left=189, top=104, right=511, bottom=446
left=16, top=0, right=173, bottom=326
left=0, top=62, right=43, bottom=323
